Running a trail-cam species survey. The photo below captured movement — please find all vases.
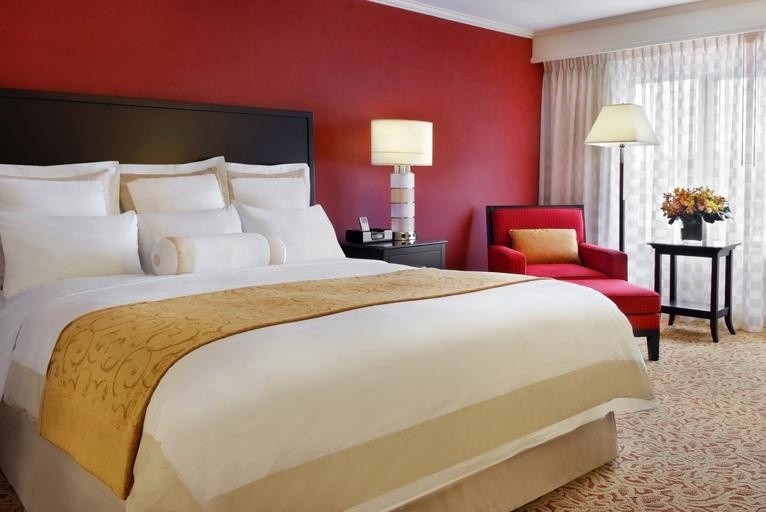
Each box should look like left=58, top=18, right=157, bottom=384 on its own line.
left=681, top=218, right=704, bottom=240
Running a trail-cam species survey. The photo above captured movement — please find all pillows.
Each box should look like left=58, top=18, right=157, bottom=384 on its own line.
left=231, top=199, right=345, bottom=266
left=136, top=202, right=241, bottom=275
left=0, top=210, right=144, bottom=300
left=0, top=160, right=118, bottom=218
left=508, top=228, right=581, bottom=266
left=225, top=163, right=312, bottom=210
left=118, top=154, right=231, bottom=212
left=151, top=230, right=285, bottom=276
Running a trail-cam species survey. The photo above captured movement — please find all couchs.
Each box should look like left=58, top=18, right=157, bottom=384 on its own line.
left=485, top=204, right=629, bottom=279
left=558, top=278, right=662, bottom=361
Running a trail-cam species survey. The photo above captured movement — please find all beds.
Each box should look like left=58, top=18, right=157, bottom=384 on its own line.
left=0, top=87, right=654, bottom=510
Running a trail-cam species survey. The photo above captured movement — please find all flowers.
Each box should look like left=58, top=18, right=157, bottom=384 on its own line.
left=662, top=186, right=734, bottom=224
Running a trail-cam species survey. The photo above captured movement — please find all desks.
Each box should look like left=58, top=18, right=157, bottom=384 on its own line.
left=647, top=239, right=742, bottom=342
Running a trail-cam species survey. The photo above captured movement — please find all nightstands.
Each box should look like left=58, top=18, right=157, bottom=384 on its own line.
left=338, top=237, right=448, bottom=270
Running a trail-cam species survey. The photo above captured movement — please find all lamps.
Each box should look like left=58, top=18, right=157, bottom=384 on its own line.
left=368, top=118, right=433, bottom=239
left=582, top=103, right=658, bottom=252
